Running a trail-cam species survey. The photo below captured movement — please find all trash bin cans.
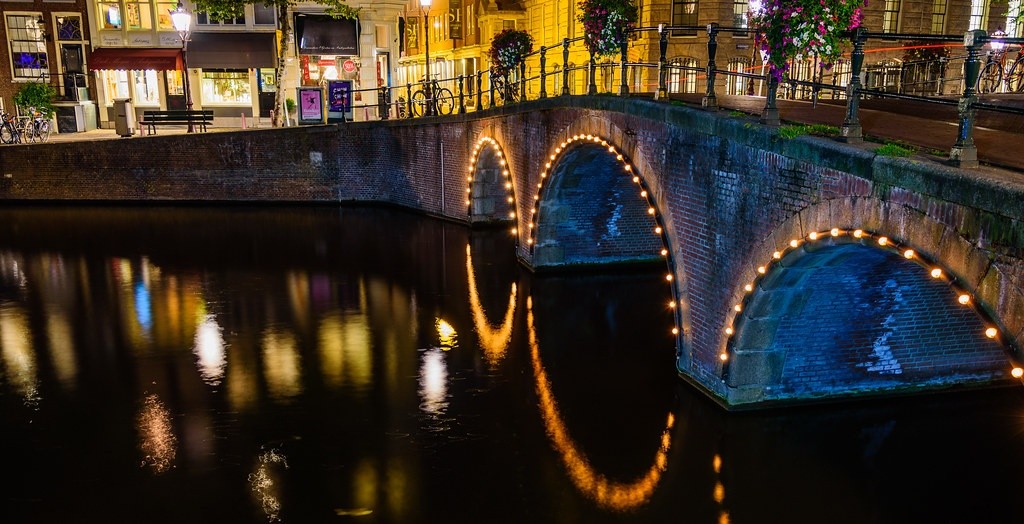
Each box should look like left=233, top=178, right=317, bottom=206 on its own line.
left=113, top=98, right=136, bottom=137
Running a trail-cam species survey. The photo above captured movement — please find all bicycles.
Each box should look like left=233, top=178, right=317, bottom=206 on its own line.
left=0, top=107, right=51, bottom=144
left=412, top=73, right=455, bottom=117
left=977, top=48, right=1024, bottom=94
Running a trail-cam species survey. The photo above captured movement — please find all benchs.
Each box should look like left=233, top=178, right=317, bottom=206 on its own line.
left=139, top=110, right=214, bottom=136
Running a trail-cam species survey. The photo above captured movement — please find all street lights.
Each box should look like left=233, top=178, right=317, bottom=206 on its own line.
left=420, top=0, right=432, bottom=117
left=167, top=0, right=197, bottom=133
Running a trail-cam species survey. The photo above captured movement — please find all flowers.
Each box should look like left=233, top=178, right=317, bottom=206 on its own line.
left=484, top=29, right=535, bottom=98
left=748, top=0, right=866, bottom=83
left=577, top=0, right=639, bottom=55
